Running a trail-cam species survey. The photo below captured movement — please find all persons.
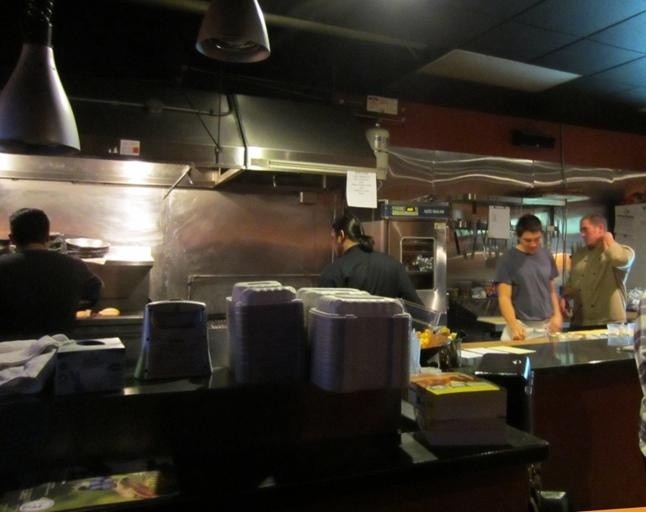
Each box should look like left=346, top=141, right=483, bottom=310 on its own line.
left=562, top=213, right=636, bottom=331
left=492, top=213, right=562, bottom=340
left=1, top=208, right=103, bottom=344
left=317, top=214, right=430, bottom=334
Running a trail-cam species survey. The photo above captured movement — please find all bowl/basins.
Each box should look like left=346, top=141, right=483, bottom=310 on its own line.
left=458, top=219, right=468, bottom=228
left=420, top=341, right=459, bottom=372
left=65, top=237, right=110, bottom=258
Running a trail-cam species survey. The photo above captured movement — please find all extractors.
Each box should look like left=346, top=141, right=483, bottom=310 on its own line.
left=0, top=57, right=378, bottom=193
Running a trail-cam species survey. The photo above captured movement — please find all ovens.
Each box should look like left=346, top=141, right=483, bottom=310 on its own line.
left=346, top=199, right=448, bottom=313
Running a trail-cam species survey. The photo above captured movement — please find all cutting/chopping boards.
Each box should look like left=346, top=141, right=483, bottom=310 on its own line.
left=460, top=335, right=549, bottom=348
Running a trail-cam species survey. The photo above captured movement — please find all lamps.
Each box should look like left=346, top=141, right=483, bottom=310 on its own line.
left=0, top=0, right=82, bottom=156
left=194, top=1, right=271, bottom=64
